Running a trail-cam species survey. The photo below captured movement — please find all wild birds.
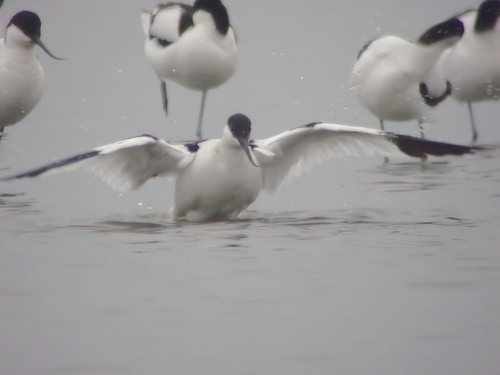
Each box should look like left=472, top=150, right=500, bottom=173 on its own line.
left=0, top=10, right=60, bottom=137
left=0, top=114, right=487, bottom=224
left=352, top=0, right=500, bottom=146
left=139, top=0, right=239, bottom=142
